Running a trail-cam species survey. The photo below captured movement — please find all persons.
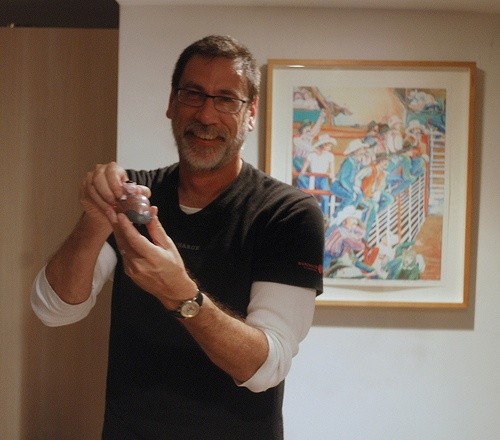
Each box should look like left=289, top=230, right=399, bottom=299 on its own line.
left=28, top=35, right=324, bottom=440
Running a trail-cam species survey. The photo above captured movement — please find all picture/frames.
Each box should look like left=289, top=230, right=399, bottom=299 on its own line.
left=266, top=58, right=476, bottom=310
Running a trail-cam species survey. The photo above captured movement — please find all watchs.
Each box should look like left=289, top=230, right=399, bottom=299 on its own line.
left=167, top=290, right=204, bottom=321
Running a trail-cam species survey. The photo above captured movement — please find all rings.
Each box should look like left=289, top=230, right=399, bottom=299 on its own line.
left=119, top=248, right=127, bottom=256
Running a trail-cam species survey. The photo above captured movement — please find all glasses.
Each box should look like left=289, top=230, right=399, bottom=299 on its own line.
left=174, top=88, right=250, bottom=115
